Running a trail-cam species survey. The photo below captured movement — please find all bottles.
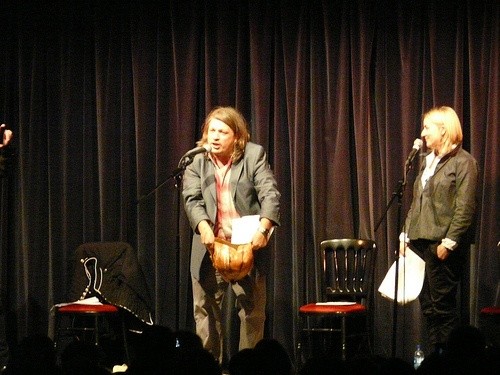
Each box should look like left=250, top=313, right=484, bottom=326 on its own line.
left=414, top=344, right=425, bottom=370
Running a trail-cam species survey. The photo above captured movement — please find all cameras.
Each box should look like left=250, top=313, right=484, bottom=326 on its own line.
left=175, top=338, right=181, bottom=347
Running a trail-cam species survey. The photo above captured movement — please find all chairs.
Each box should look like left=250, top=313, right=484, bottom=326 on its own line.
left=55, top=242, right=135, bottom=369
left=297, top=237, right=378, bottom=363
left=479, top=241, right=500, bottom=350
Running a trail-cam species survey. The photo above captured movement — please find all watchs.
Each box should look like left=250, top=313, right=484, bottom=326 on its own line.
left=258, top=226, right=270, bottom=238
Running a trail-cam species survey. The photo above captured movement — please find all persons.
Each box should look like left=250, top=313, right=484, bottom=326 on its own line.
left=398, top=106, right=481, bottom=364
left=0, top=321, right=500, bottom=375
left=182, top=107, right=282, bottom=367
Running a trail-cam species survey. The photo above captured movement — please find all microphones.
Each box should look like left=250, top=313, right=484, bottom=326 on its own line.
left=405, top=139, right=423, bottom=165
left=178, top=143, right=212, bottom=168
